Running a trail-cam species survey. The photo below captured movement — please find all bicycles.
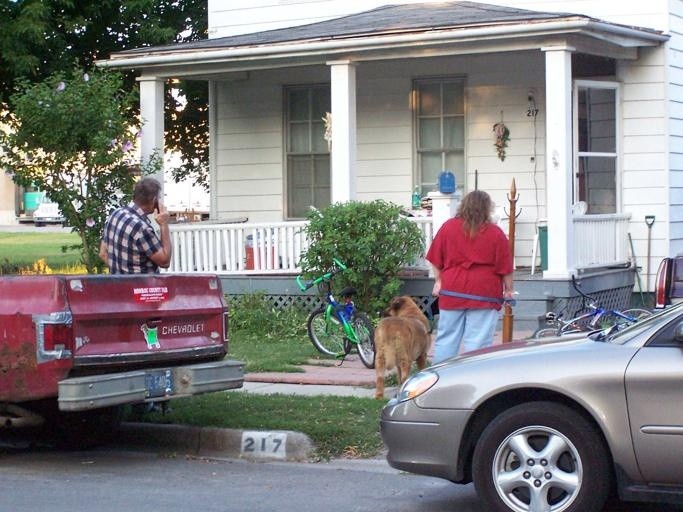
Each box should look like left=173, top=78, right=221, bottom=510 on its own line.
left=294, top=259, right=376, bottom=370
left=531, top=273, right=653, bottom=338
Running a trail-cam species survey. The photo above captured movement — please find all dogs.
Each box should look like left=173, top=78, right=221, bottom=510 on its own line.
left=374, top=295, right=431, bottom=401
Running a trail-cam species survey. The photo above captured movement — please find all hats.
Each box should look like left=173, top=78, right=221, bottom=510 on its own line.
left=440, top=173, right=455, bottom=193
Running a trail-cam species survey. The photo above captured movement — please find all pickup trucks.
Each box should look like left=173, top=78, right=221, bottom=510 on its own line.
left=0, top=269, right=248, bottom=449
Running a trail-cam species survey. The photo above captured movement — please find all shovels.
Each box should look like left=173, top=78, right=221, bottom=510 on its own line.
left=642, top=216, right=657, bottom=308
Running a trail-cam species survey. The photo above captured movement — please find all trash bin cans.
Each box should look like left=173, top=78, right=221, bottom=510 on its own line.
left=538, top=226, right=548, bottom=272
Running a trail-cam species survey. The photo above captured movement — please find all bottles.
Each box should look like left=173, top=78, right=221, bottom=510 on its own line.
left=412, top=184, right=422, bottom=211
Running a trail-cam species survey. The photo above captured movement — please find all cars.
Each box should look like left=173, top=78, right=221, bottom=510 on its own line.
left=381, top=298, right=682, bottom=512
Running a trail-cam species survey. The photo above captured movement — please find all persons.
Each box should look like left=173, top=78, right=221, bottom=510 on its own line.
left=426, top=190, right=519, bottom=367
left=97, top=178, right=173, bottom=415
